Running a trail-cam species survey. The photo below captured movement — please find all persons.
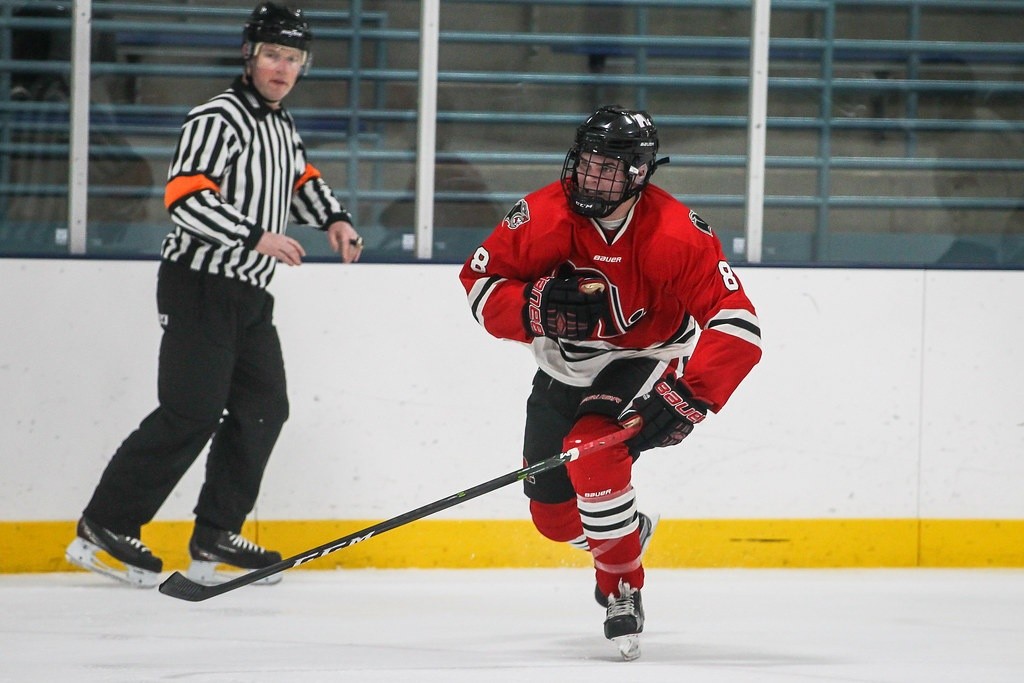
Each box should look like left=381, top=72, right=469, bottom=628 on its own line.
left=460, top=105, right=763, bottom=662
left=8, top=1, right=156, bottom=245
left=66, top=0, right=363, bottom=587
left=382, top=92, right=505, bottom=226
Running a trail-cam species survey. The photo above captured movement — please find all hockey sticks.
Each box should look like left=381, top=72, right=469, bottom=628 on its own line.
left=158, top=420, right=644, bottom=604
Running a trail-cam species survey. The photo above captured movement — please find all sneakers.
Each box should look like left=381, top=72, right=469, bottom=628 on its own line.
left=64, top=515, right=163, bottom=587
left=187, top=517, right=282, bottom=585
left=604, top=577, right=645, bottom=661
left=594, top=511, right=660, bottom=608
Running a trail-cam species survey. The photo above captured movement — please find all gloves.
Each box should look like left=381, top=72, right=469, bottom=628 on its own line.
left=520, top=262, right=606, bottom=339
left=611, top=375, right=707, bottom=451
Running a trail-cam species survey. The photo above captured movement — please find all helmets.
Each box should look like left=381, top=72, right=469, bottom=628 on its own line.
left=242, top=2, right=312, bottom=76
left=566, top=103, right=670, bottom=218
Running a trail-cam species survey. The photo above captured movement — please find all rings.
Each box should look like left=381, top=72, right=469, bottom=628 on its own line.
left=350, top=238, right=364, bottom=248
